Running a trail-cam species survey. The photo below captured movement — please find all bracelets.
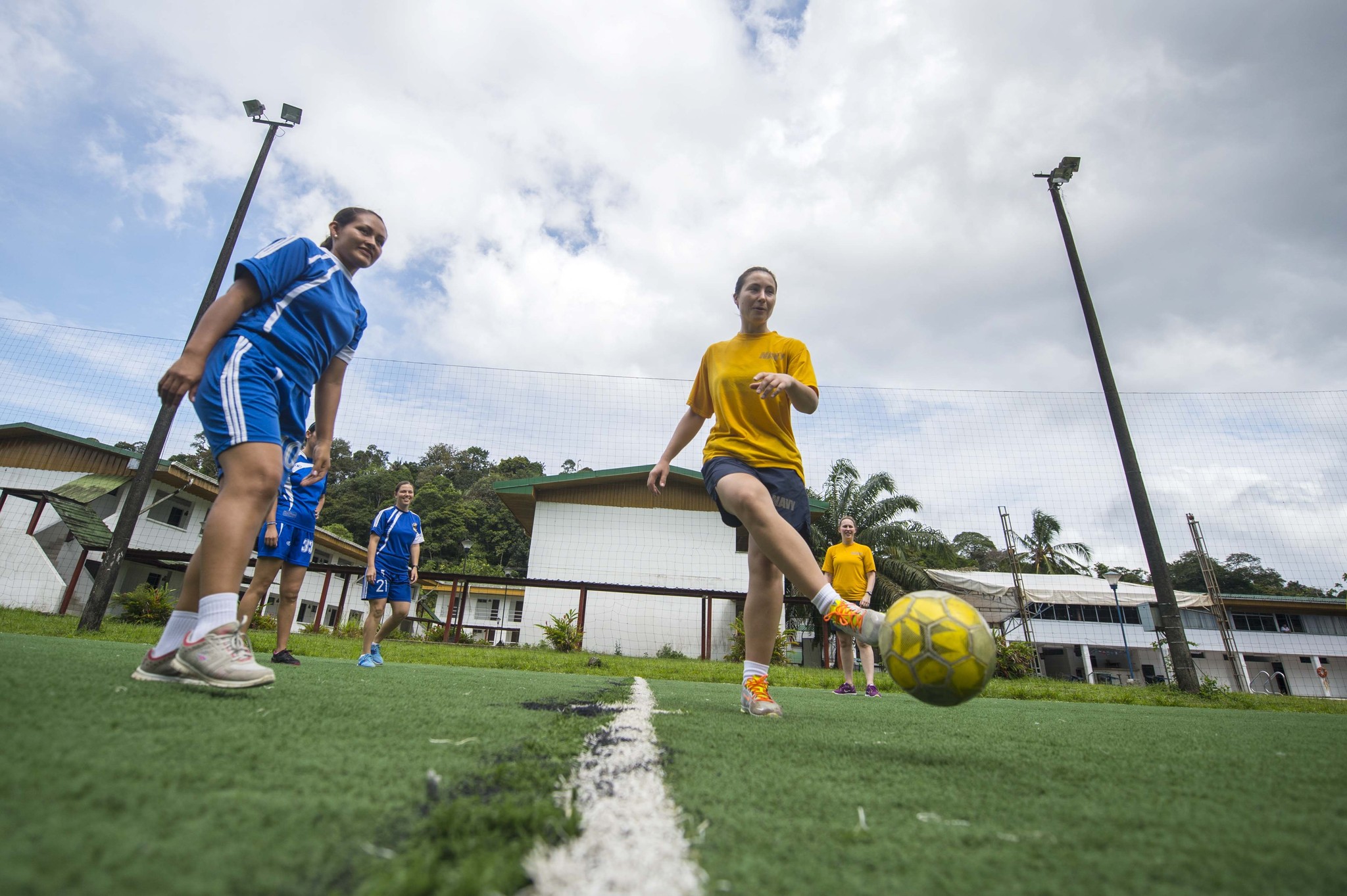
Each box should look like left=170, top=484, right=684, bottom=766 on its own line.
left=266, top=521, right=277, bottom=526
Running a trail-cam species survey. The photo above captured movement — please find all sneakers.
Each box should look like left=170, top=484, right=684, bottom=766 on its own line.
left=865, top=685, right=882, bottom=697
left=371, top=643, right=384, bottom=665
left=826, top=602, right=885, bottom=646
left=169, top=620, right=275, bottom=688
left=131, top=648, right=209, bottom=686
left=357, top=654, right=375, bottom=667
left=271, top=649, right=300, bottom=666
left=831, top=683, right=856, bottom=695
left=741, top=677, right=782, bottom=717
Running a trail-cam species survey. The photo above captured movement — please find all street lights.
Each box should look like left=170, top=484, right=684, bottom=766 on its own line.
left=1034, top=156, right=1201, bottom=694
left=78, top=100, right=304, bottom=632
left=1102, top=571, right=1135, bottom=680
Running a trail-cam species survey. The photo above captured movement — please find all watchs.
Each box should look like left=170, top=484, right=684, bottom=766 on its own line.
left=865, top=591, right=873, bottom=596
left=412, top=564, right=420, bottom=570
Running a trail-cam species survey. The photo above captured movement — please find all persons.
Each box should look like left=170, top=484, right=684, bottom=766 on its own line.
left=129, top=199, right=389, bottom=693
left=644, top=265, right=889, bottom=721
left=231, top=421, right=330, bottom=667
left=821, top=517, right=883, bottom=702
left=1281, top=624, right=1291, bottom=633
left=356, top=480, right=425, bottom=668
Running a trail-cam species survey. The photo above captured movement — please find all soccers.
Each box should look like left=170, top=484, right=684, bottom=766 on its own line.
left=876, top=590, right=999, bottom=708
